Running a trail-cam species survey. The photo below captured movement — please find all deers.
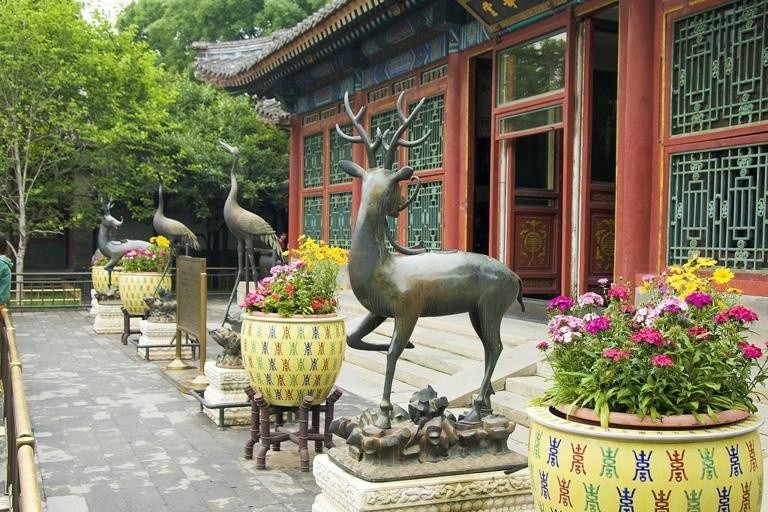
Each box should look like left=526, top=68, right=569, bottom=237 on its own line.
left=335, top=91, right=526, bottom=431
left=97, top=192, right=159, bottom=290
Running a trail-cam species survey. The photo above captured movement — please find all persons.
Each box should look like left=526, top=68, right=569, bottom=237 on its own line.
left=0, top=236, right=14, bottom=304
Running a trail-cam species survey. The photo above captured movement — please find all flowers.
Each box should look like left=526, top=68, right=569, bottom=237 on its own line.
left=91, top=236, right=175, bottom=272
left=237, top=231, right=351, bottom=310
left=526, top=244, right=768, bottom=422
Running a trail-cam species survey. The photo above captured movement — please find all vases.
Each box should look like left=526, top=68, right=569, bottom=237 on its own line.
left=89, top=266, right=174, bottom=316
left=521, top=406, right=764, bottom=512
left=237, top=316, right=345, bottom=406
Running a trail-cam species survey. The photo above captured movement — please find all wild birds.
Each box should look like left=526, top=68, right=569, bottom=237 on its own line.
left=219, top=141, right=285, bottom=328
left=152, top=171, right=202, bottom=297
